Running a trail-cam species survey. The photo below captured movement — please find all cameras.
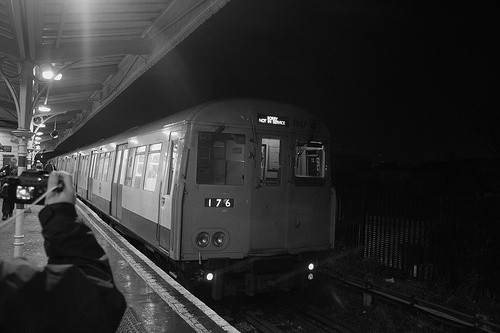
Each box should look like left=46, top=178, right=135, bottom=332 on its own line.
left=8, top=171, right=64, bottom=207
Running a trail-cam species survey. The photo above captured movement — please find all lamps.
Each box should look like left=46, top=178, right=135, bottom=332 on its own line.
left=31, top=67, right=63, bottom=152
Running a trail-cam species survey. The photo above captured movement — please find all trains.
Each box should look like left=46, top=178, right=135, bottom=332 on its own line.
left=43, top=98, right=337, bottom=301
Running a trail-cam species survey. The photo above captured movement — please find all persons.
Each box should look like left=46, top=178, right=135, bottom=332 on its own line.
left=1, top=162, right=60, bottom=219
left=0, top=170, right=127, bottom=332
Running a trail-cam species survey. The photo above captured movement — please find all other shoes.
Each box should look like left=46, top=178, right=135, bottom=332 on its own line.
left=2, top=213, right=8, bottom=221
left=9, top=213, right=13, bottom=218
